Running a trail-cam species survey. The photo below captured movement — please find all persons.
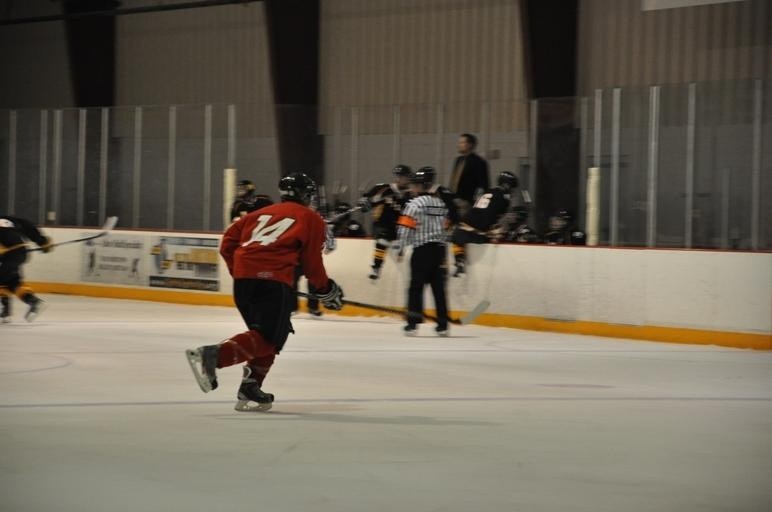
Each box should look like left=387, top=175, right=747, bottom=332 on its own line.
left=395, top=172, right=448, bottom=334
left=188, top=171, right=344, bottom=413
left=230, top=179, right=274, bottom=223
left=0, top=215, right=53, bottom=326
left=319, top=133, right=588, bottom=281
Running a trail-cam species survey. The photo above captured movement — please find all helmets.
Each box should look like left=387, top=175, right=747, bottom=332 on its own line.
left=392, top=164, right=519, bottom=188
left=237, top=180, right=256, bottom=198
left=279, top=172, right=316, bottom=201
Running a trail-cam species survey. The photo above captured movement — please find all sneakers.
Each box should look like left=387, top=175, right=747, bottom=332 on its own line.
left=199, top=345, right=218, bottom=390
left=370, top=261, right=466, bottom=330
left=0, top=297, right=11, bottom=317
left=21, top=292, right=43, bottom=318
left=238, top=380, right=273, bottom=403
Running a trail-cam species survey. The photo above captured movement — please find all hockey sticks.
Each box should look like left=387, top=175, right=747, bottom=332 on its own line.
left=297, top=291, right=491, bottom=326
left=27, top=217, right=118, bottom=252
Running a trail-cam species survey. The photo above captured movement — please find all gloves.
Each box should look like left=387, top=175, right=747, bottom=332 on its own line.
left=315, top=280, right=344, bottom=310
